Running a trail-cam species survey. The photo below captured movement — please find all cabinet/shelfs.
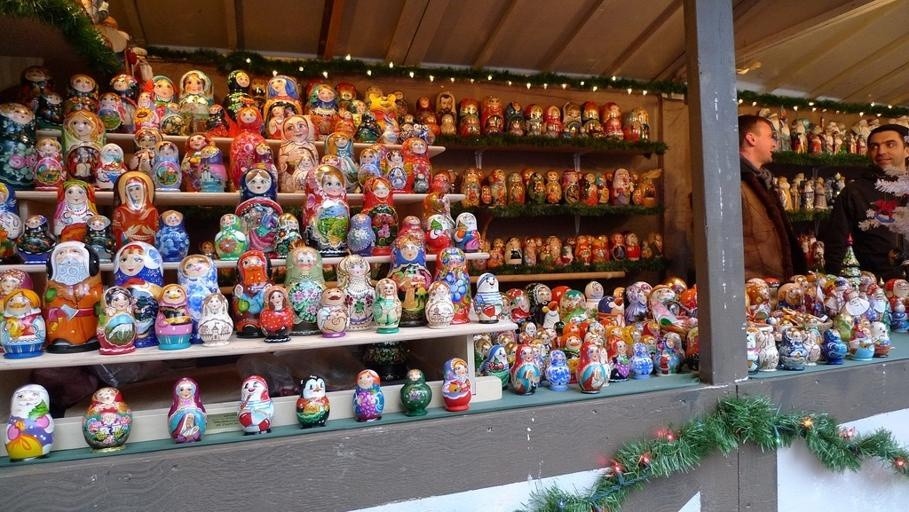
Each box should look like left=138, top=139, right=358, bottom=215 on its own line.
left=0, top=77, right=519, bottom=472
left=411, top=95, right=668, bottom=285
left=740, top=109, right=909, bottom=276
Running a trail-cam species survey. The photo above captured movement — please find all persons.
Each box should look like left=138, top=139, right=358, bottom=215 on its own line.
left=816, top=123, right=908, bottom=285
left=738, top=115, right=807, bottom=282
